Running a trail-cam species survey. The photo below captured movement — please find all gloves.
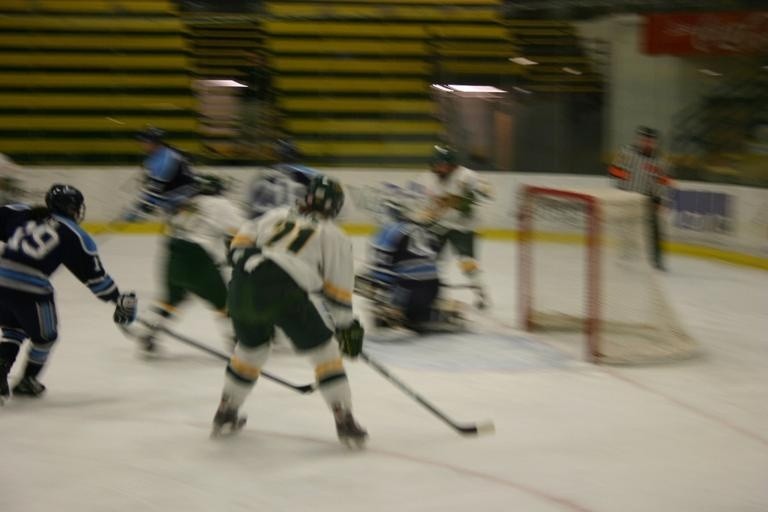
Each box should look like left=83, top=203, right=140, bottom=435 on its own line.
left=334, top=319, right=364, bottom=357
left=113, top=292, right=138, bottom=324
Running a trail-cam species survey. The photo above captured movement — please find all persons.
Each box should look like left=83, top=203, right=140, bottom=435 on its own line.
left=1, top=183, right=138, bottom=398
left=211, top=174, right=369, bottom=446
left=127, top=128, right=196, bottom=223
left=609, top=125, right=668, bottom=271
left=370, top=145, right=492, bottom=334
left=138, top=164, right=314, bottom=356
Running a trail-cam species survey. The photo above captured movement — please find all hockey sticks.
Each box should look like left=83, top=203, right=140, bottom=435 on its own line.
left=134, top=315, right=319, bottom=395
left=360, top=351, right=496, bottom=436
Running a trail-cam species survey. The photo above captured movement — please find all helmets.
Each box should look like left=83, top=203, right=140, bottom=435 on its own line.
left=45, top=185, right=84, bottom=217
left=305, top=174, right=345, bottom=218
left=430, top=145, right=456, bottom=174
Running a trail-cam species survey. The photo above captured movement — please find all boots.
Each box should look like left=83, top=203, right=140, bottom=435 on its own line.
left=0, top=377, right=46, bottom=397
left=336, top=417, right=368, bottom=439
left=210, top=413, right=248, bottom=437
